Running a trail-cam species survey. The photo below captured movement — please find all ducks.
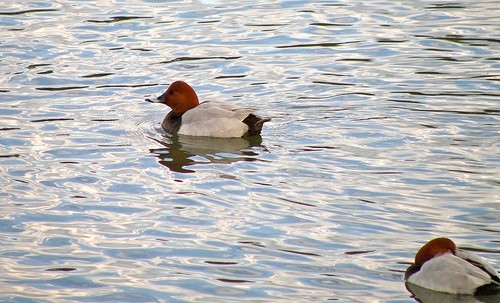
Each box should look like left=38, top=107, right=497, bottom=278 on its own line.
left=405, top=237, right=500, bottom=296
left=145, top=80, right=272, bottom=138
